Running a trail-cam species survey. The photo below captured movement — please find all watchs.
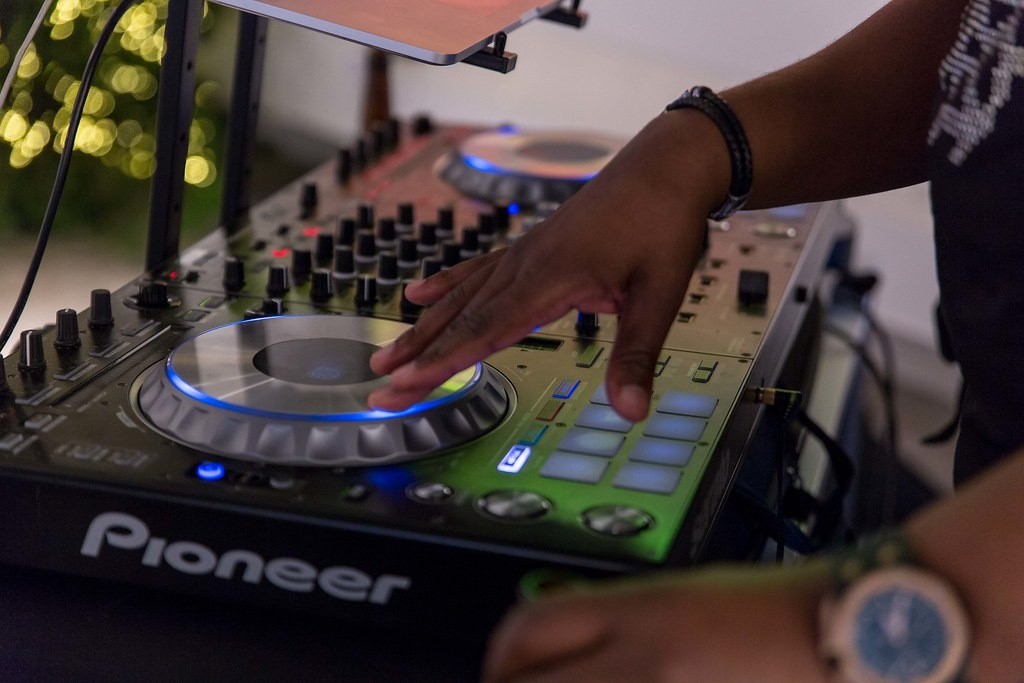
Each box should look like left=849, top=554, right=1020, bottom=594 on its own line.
left=815, top=522, right=974, bottom=681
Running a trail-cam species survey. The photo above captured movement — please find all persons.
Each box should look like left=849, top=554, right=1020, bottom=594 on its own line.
left=367, top=1, right=1024, bottom=683
